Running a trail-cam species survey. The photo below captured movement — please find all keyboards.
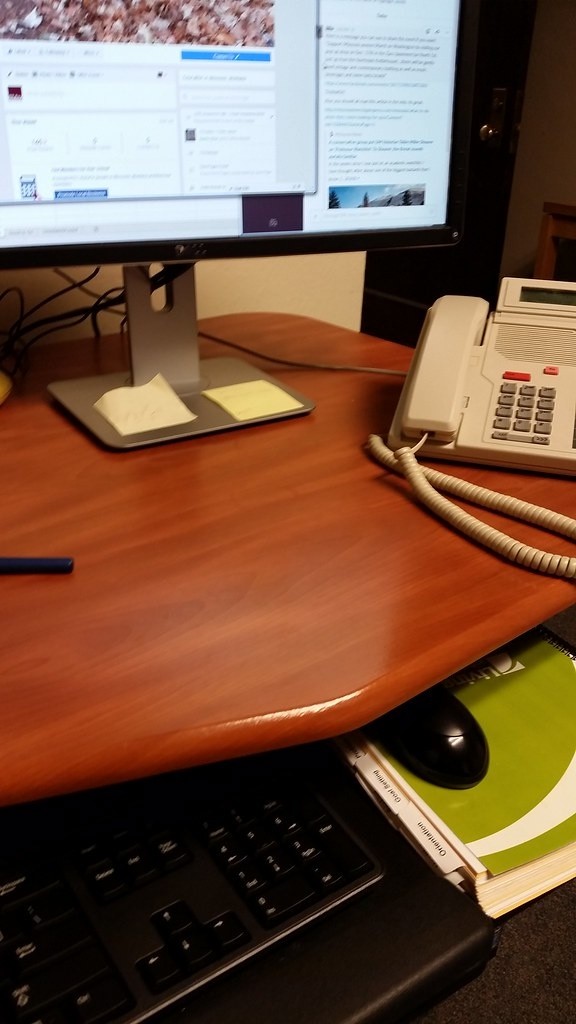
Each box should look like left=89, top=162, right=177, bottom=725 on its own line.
left=0, top=751, right=385, bottom=1024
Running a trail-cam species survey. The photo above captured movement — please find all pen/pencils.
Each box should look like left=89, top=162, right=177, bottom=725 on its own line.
left=0, top=558, right=74, bottom=574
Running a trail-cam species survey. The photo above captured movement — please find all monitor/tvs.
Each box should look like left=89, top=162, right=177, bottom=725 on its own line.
left=0, top=0, right=480, bottom=451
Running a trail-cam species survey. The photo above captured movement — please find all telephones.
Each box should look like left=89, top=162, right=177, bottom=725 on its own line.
left=387, top=276, right=576, bottom=479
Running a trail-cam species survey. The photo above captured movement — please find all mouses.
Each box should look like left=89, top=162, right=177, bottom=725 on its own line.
left=366, top=683, right=489, bottom=790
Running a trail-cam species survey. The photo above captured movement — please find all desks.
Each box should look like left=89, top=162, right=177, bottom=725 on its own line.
left=0, top=310, right=576, bottom=808
